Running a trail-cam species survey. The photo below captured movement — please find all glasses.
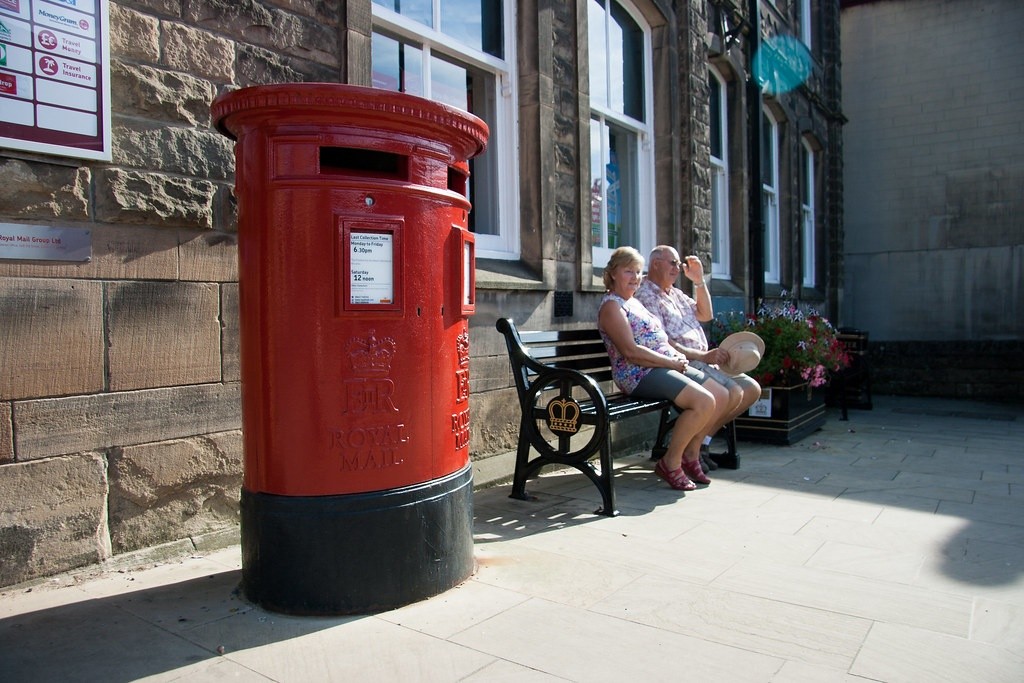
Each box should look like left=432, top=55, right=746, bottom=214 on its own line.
left=657, top=258, right=683, bottom=267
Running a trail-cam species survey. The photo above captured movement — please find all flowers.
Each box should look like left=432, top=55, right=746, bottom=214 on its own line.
left=707, top=289, right=854, bottom=401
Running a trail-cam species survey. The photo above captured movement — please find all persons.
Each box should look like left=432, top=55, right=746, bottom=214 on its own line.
left=631, top=245, right=762, bottom=474
left=596, top=246, right=731, bottom=490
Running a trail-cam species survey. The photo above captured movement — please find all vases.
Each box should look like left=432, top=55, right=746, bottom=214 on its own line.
left=718, top=382, right=827, bottom=445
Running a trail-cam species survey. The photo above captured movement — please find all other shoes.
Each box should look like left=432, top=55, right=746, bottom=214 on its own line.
left=703, top=455, right=718, bottom=470
left=699, top=459, right=709, bottom=474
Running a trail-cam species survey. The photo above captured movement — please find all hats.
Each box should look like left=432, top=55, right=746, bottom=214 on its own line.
left=717, top=331, right=765, bottom=374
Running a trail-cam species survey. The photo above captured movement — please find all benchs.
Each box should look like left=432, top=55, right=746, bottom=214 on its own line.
left=495, top=316, right=740, bottom=517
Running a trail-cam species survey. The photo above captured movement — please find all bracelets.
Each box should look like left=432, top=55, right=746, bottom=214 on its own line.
left=695, top=279, right=706, bottom=288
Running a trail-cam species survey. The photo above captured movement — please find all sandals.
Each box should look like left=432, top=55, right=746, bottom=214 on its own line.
left=681, top=454, right=711, bottom=484
left=655, top=458, right=697, bottom=491
